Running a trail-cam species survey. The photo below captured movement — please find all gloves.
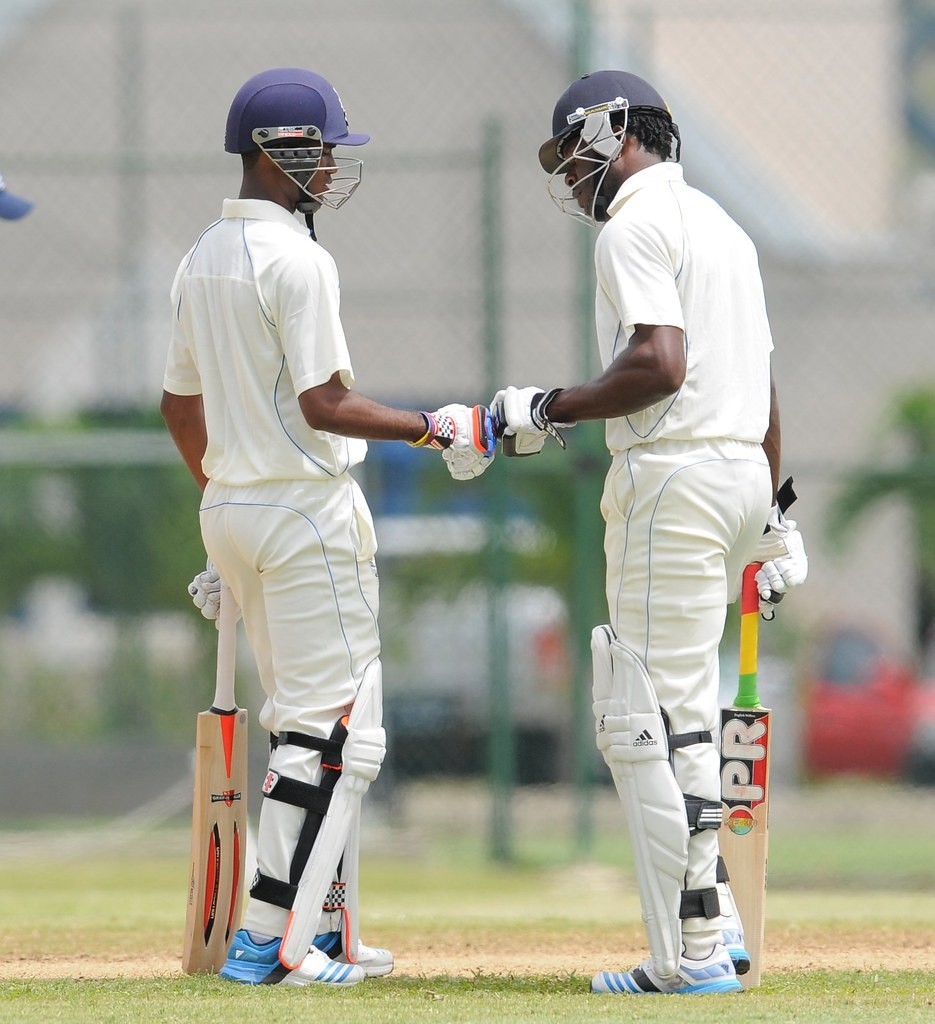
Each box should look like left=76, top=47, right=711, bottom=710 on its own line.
left=407, top=404, right=497, bottom=480
left=489, top=386, right=578, bottom=457
left=753, top=502, right=808, bottom=622
left=187, top=554, right=242, bottom=632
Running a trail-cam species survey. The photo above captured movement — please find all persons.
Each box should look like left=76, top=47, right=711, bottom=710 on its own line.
left=492, top=69, right=807, bottom=995
left=160, top=68, right=494, bottom=986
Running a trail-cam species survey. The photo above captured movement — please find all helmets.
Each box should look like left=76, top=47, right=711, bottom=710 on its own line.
left=539, top=67, right=673, bottom=174
left=223, top=67, right=371, bottom=154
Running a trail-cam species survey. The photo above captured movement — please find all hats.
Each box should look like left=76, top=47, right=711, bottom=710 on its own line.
left=0, top=176, right=34, bottom=220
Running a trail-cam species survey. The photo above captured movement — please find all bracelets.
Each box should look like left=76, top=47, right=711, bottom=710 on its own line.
left=404, top=409, right=453, bottom=451
left=537, top=387, right=578, bottom=449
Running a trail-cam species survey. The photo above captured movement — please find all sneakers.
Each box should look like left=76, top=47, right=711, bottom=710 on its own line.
left=589, top=941, right=742, bottom=995
left=721, top=929, right=750, bottom=974
left=219, top=928, right=364, bottom=988
left=311, top=927, right=394, bottom=978
